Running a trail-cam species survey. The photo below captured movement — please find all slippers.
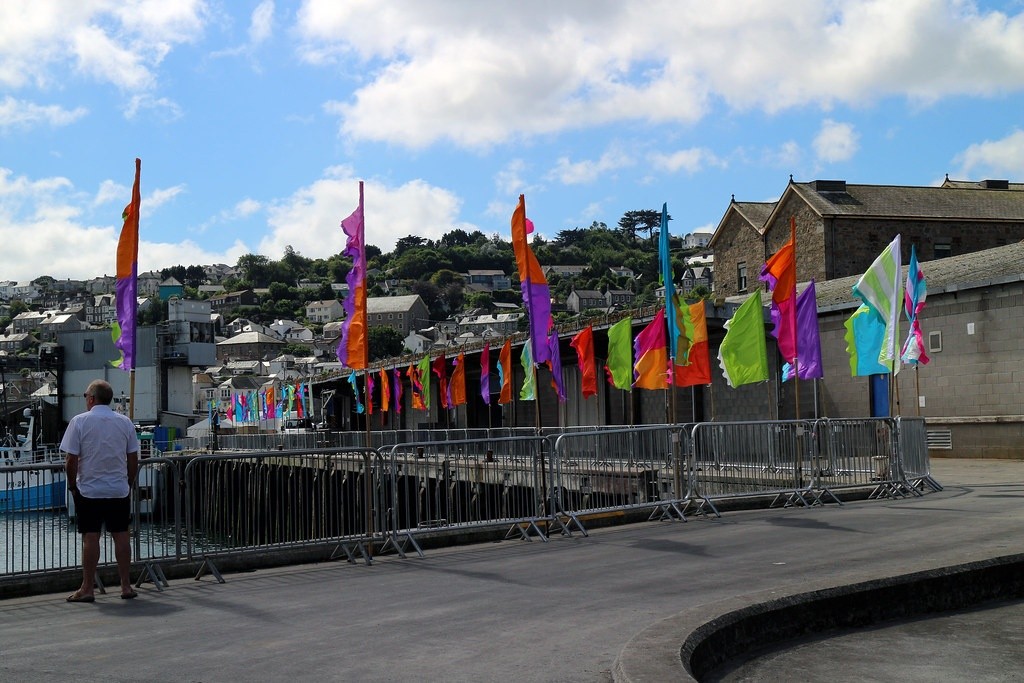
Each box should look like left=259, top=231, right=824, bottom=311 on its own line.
left=121, top=586, right=137, bottom=599
left=66, top=590, right=95, bottom=602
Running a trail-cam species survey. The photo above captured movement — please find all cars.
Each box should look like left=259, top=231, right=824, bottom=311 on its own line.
left=315, top=421, right=327, bottom=429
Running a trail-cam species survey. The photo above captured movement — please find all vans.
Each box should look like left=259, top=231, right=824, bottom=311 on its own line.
left=283, top=410, right=303, bottom=428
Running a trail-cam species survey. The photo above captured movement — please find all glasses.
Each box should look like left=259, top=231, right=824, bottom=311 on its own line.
left=84, top=392, right=89, bottom=398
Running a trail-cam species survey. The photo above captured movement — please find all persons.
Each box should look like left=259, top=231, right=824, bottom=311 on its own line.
left=60, top=379, right=139, bottom=602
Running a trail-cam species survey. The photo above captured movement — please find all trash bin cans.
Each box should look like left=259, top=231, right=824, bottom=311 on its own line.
left=155, top=426, right=180, bottom=452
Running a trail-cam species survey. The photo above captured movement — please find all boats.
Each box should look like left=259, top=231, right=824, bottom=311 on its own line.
left=64, top=392, right=166, bottom=527
left=0, top=408, right=67, bottom=514
